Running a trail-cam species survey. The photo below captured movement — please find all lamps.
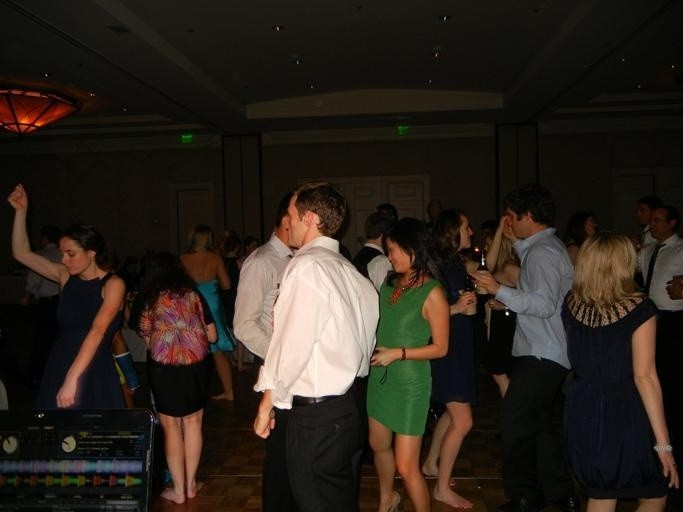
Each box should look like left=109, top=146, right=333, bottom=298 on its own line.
left=0, top=81, right=80, bottom=135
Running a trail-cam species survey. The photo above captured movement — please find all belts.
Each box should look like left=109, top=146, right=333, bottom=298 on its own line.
left=293, top=395, right=333, bottom=405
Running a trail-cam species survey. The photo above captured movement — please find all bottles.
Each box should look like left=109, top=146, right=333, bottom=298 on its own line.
left=476, top=250, right=490, bottom=298
left=462, top=273, right=476, bottom=318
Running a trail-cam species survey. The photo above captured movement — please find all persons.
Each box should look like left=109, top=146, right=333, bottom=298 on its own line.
left=231, top=181, right=682, bottom=510
left=7, top=184, right=260, bottom=505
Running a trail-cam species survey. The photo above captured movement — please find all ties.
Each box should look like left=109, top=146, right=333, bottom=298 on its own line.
left=645, top=244, right=665, bottom=295
